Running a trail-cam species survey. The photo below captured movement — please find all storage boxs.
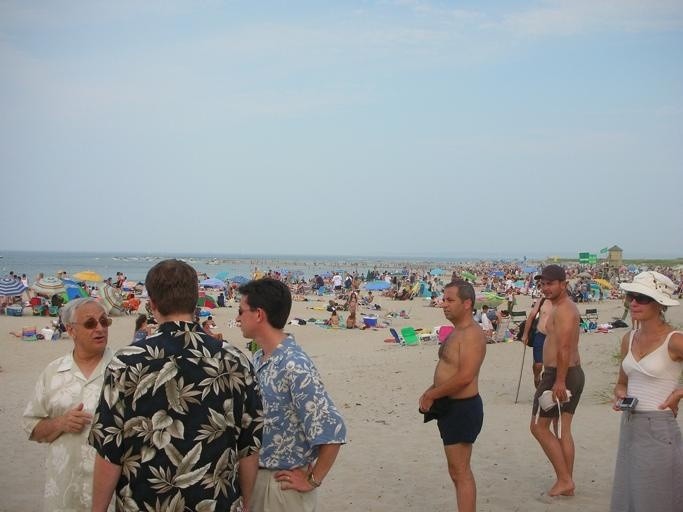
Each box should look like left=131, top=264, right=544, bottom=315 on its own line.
left=5, top=306, right=22, bottom=317
left=361, top=316, right=378, bottom=326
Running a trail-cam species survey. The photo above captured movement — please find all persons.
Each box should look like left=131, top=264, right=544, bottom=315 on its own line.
left=530, top=265, right=587, bottom=497
left=86, top=259, right=265, bottom=512
left=235, top=278, right=347, bottom=511
left=20, top=297, right=117, bottom=511
left=0, top=256, right=683, bottom=344
left=610, top=269, right=683, bottom=512
left=416, top=280, right=488, bottom=512
left=522, top=296, right=552, bottom=389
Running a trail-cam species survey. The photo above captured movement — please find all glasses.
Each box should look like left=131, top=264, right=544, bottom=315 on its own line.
left=70, top=316, right=113, bottom=330
left=238, top=305, right=258, bottom=317
left=625, top=291, right=656, bottom=305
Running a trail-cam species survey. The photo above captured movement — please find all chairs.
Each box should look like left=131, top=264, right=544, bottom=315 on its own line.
left=122, top=300, right=141, bottom=317
left=582, top=306, right=598, bottom=324
left=51, top=316, right=67, bottom=341
left=388, top=325, right=403, bottom=346
left=401, top=325, right=423, bottom=352
left=484, top=316, right=510, bottom=343
left=510, top=310, right=527, bottom=324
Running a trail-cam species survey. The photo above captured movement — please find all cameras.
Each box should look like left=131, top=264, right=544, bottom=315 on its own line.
left=616, top=396, right=638, bottom=409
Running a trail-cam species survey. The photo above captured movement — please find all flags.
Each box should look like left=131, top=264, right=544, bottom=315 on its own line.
left=599, top=247, right=607, bottom=254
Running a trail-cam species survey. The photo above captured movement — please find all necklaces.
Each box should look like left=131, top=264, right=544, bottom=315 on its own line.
left=636, top=336, right=664, bottom=358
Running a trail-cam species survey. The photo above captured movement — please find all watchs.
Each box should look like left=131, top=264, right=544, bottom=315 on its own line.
left=306, top=472, right=322, bottom=488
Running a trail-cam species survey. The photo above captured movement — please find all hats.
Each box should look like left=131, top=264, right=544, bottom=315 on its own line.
left=619, top=270, right=681, bottom=309
left=533, top=264, right=567, bottom=282
